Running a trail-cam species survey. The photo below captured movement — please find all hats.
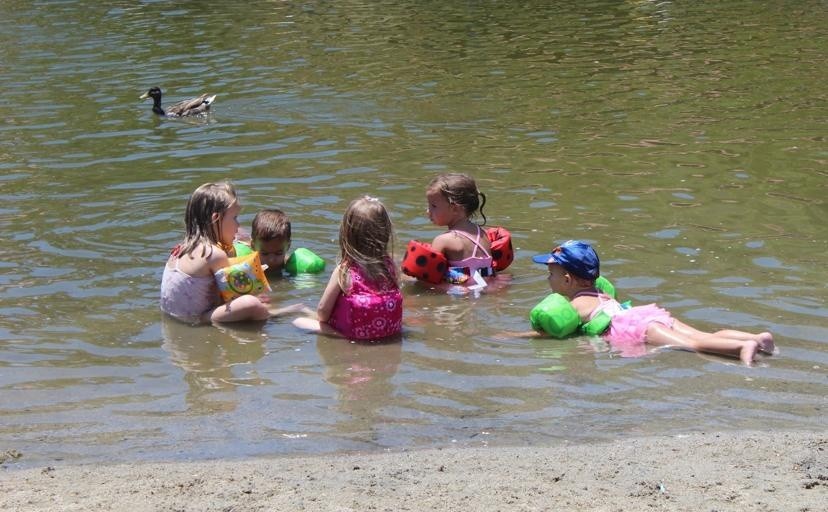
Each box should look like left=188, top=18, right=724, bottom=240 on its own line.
left=531, top=240, right=600, bottom=279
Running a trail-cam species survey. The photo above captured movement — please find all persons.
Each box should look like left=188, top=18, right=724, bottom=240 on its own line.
left=295, top=194, right=403, bottom=341
left=160, top=183, right=304, bottom=325
left=234, top=208, right=325, bottom=275
left=425, top=173, right=496, bottom=292
left=495, top=239, right=775, bottom=367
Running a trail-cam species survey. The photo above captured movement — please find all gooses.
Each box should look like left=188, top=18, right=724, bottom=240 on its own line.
left=139, top=86, right=217, bottom=117
